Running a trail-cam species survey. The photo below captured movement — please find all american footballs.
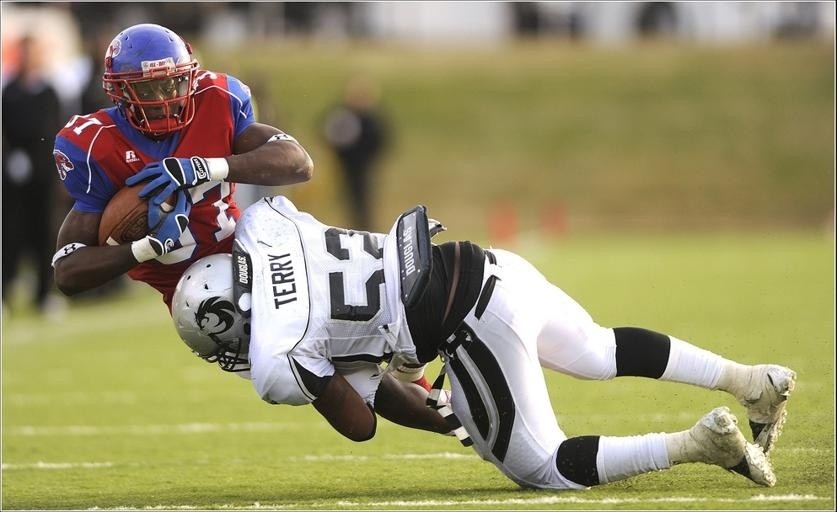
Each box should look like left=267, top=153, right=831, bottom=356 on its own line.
left=99, top=179, right=178, bottom=246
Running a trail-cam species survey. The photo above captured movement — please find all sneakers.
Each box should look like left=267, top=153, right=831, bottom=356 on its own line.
left=689, top=364, right=796, bottom=487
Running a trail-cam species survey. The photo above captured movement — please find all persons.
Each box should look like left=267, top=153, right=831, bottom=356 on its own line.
left=325, top=71, right=397, bottom=230
left=171, top=194, right=797, bottom=490
left=51, top=21, right=460, bottom=440
left=1, top=32, right=128, bottom=310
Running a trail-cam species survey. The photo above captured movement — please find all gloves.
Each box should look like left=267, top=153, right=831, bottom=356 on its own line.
left=126, top=156, right=228, bottom=264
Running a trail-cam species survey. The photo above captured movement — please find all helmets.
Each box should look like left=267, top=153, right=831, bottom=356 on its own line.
left=105, top=23, right=193, bottom=142
left=172, top=253, right=252, bottom=359
